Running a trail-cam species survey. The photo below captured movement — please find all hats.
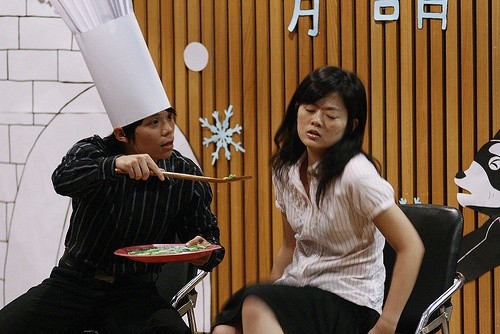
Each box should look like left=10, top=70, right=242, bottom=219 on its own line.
left=49, top=0, right=171, bottom=129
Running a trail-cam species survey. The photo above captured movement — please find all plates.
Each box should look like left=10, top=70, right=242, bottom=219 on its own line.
left=114, top=243, right=223, bottom=263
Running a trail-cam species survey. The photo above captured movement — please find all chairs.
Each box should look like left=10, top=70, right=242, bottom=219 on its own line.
left=171, top=269, right=209, bottom=334
left=383, top=204, right=465, bottom=334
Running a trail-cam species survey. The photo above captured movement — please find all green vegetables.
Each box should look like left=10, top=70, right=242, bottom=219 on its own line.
left=125, top=244, right=205, bottom=255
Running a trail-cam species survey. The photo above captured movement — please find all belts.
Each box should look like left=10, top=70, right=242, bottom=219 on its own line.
left=61, top=253, right=161, bottom=283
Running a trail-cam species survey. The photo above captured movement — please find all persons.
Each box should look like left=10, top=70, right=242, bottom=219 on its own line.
left=0, top=86, right=225, bottom=334
left=211, top=66, right=424, bottom=334
